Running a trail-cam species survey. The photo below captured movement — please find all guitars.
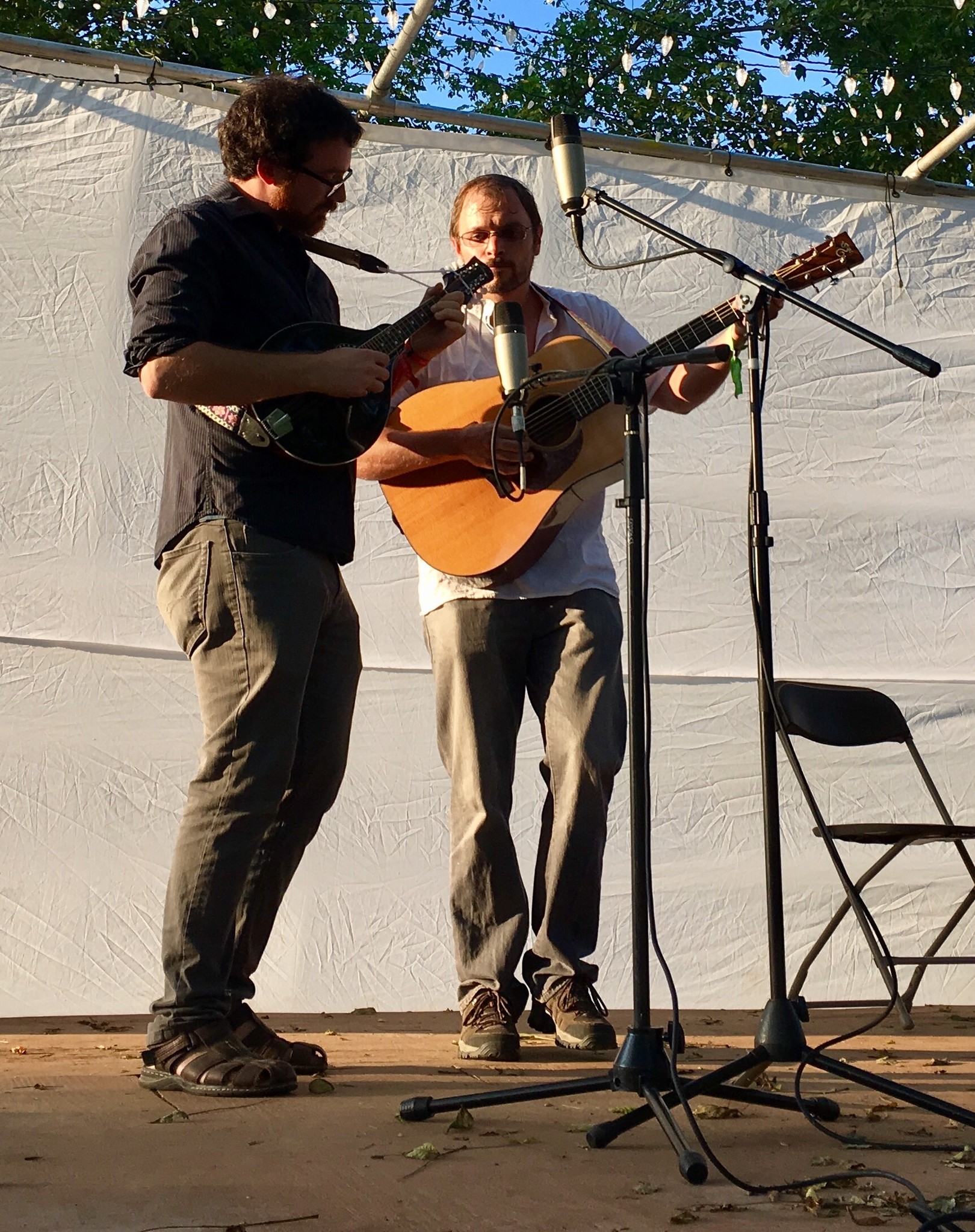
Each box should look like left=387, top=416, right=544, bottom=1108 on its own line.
left=378, top=230, right=865, bottom=590
left=250, top=255, right=495, bottom=468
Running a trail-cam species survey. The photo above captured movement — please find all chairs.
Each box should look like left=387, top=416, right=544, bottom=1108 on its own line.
left=766, top=680, right=975, bottom=1029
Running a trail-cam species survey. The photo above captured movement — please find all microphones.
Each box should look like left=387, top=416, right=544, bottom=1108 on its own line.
left=550, top=113, right=586, bottom=249
left=491, top=300, right=529, bottom=437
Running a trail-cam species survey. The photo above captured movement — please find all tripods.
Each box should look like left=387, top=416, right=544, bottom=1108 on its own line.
left=400, top=312, right=975, bottom=1184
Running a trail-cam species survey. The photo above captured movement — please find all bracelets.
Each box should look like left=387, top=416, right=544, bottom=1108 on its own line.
left=399, top=338, right=430, bottom=388
left=722, top=322, right=748, bottom=399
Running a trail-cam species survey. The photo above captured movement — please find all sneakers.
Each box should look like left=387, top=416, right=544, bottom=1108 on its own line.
left=526, top=977, right=617, bottom=1049
left=457, top=987, right=521, bottom=1060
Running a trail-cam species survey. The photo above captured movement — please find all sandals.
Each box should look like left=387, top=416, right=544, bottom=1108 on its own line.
left=138, top=1021, right=298, bottom=1097
left=220, top=1003, right=328, bottom=1075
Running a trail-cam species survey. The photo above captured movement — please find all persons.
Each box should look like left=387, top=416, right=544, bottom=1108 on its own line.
left=123, top=79, right=467, bottom=1098
left=356, top=173, right=785, bottom=1061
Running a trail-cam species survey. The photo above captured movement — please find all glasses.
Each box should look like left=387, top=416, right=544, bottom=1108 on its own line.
left=274, top=163, right=352, bottom=197
left=458, top=224, right=535, bottom=245
